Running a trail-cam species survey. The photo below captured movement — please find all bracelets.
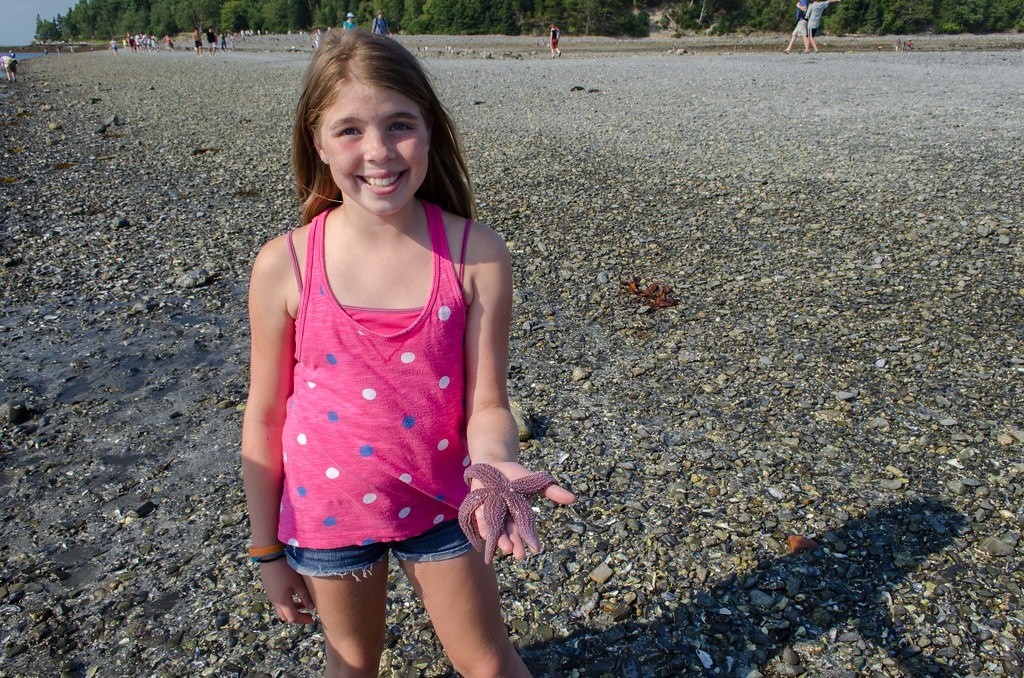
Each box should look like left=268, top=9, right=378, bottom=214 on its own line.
left=249, top=545, right=283, bottom=562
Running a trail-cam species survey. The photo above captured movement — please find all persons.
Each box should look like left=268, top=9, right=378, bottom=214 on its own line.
left=206, top=27, right=307, bottom=56
left=0, top=50, right=18, bottom=83
left=313, top=27, right=331, bottom=50
left=69, top=44, right=73, bottom=54
left=242, top=29, right=576, bottom=678
left=193, top=28, right=204, bottom=57
left=56, top=44, right=62, bottom=55
left=550, top=24, right=562, bottom=58
left=110, top=38, right=118, bottom=56
left=782, top=0, right=841, bottom=54
left=372, top=10, right=393, bottom=37
left=122, top=32, right=173, bottom=52
left=343, top=13, right=356, bottom=31
left=44, top=48, right=48, bottom=56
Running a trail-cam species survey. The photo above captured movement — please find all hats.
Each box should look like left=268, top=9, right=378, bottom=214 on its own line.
left=347, top=12, right=355, bottom=18
left=377, top=10, right=384, bottom=15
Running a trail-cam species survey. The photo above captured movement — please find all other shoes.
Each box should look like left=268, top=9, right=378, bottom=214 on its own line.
left=210, top=54, right=215, bottom=56
left=552, top=56, right=555, bottom=59
left=221, top=51, right=229, bottom=53
left=8, top=78, right=17, bottom=83
left=801, top=51, right=809, bottom=54
left=196, top=55, right=204, bottom=57
left=558, top=51, right=561, bottom=57
left=782, top=49, right=790, bottom=54
left=814, top=50, right=819, bottom=54
